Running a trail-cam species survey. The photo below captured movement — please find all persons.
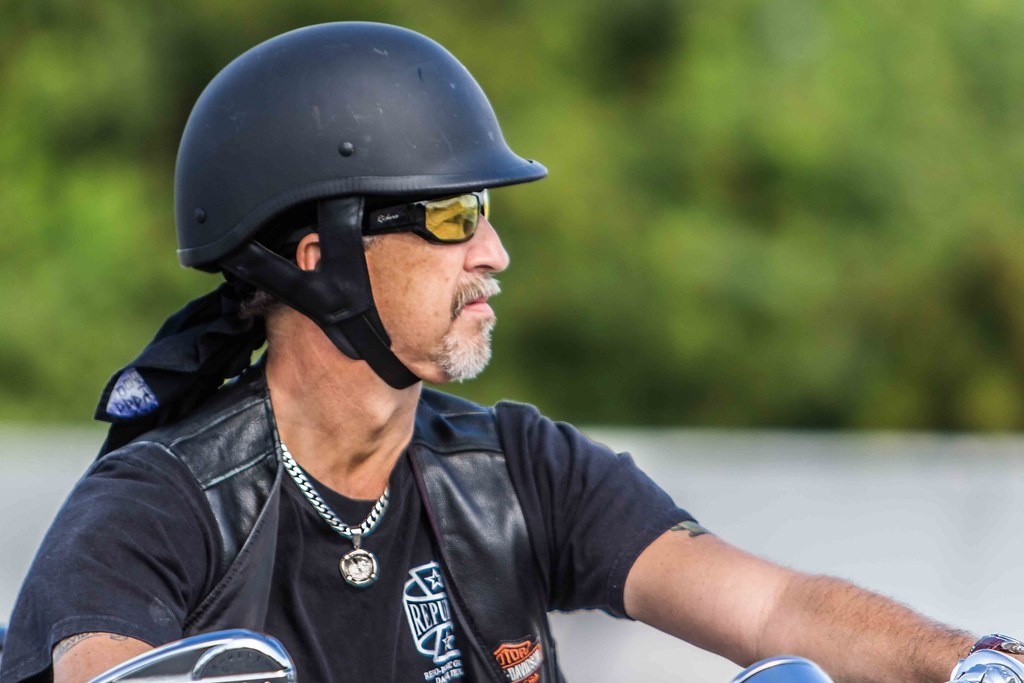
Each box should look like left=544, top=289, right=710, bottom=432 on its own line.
left=0, top=22, right=1024, bottom=683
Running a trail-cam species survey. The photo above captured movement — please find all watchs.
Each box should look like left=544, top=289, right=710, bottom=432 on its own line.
left=951, top=631, right=1024, bottom=683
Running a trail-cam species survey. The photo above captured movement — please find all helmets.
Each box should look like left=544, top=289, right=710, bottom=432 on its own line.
left=173, top=21, right=549, bottom=267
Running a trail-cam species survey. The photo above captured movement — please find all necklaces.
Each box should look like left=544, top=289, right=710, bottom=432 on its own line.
left=273, top=434, right=397, bottom=588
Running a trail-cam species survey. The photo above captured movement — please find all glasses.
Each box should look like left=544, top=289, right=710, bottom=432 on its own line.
left=354, top=189, right=491, bottom=246
